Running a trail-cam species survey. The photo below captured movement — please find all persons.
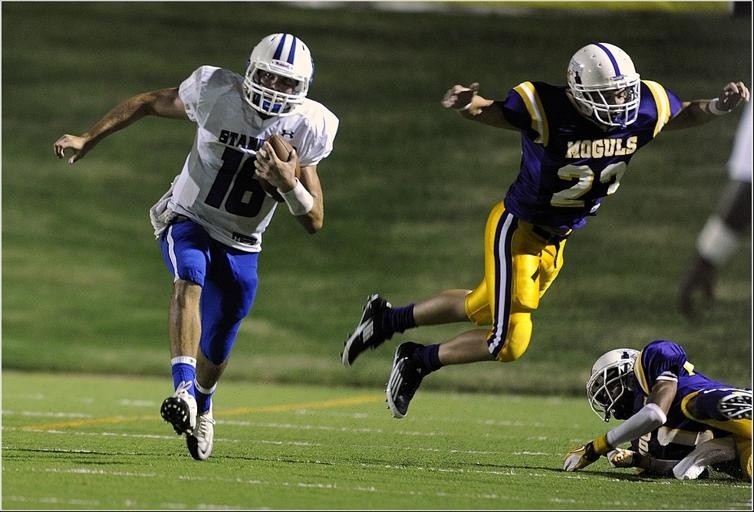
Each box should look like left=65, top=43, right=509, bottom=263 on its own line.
left=562, top=340, right=752, bottom=486
left=673, top=96, right=753, bottom=322
left=340, top=41, right=751, bottom=419
left=52, top=33, right=341, bottom=463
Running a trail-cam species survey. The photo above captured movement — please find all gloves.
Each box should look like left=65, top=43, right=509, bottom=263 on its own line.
left=563, top=432, right=616, bottom=472
left=605, top=447, right=656, bottom=472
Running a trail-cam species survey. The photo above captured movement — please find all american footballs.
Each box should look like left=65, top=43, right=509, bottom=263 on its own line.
left=255, top=135, right=301, bottom=202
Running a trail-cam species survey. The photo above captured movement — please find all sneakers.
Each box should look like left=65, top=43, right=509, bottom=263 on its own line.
left=160, top=380, right=199, bottom=437
left=185, top=396, right=216, bottom=462
left=384, top=341, right=425, bottom=420
left=339, top=292, right=392, bottom=369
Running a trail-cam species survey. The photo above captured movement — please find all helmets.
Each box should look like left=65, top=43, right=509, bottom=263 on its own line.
left=585, top=347, right=642, bottom=423
left=565, top=41, right=642, bottom=128
left=242, top=33, right=316, bottom=118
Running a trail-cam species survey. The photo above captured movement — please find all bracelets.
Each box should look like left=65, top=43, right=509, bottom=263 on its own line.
left=708, top=98, right=735, bottom=116
